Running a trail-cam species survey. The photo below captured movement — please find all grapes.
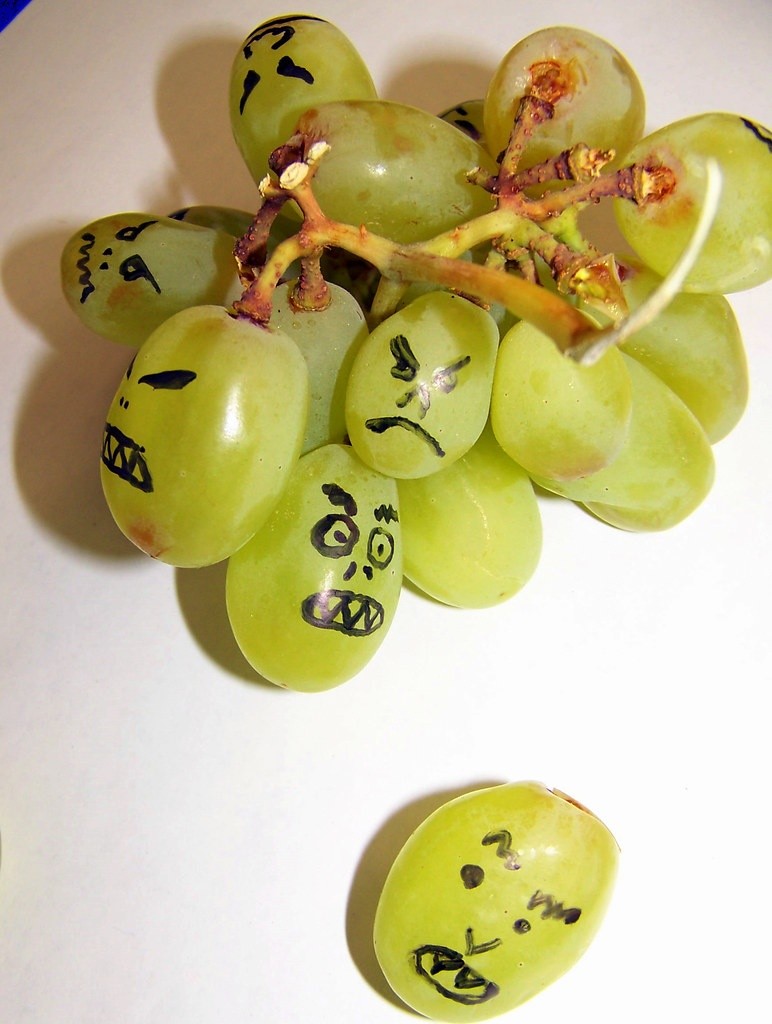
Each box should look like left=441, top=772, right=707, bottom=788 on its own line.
left=372, top=781, right=621, bottom=1024
left=55, top=14, right=771, bottom=690
